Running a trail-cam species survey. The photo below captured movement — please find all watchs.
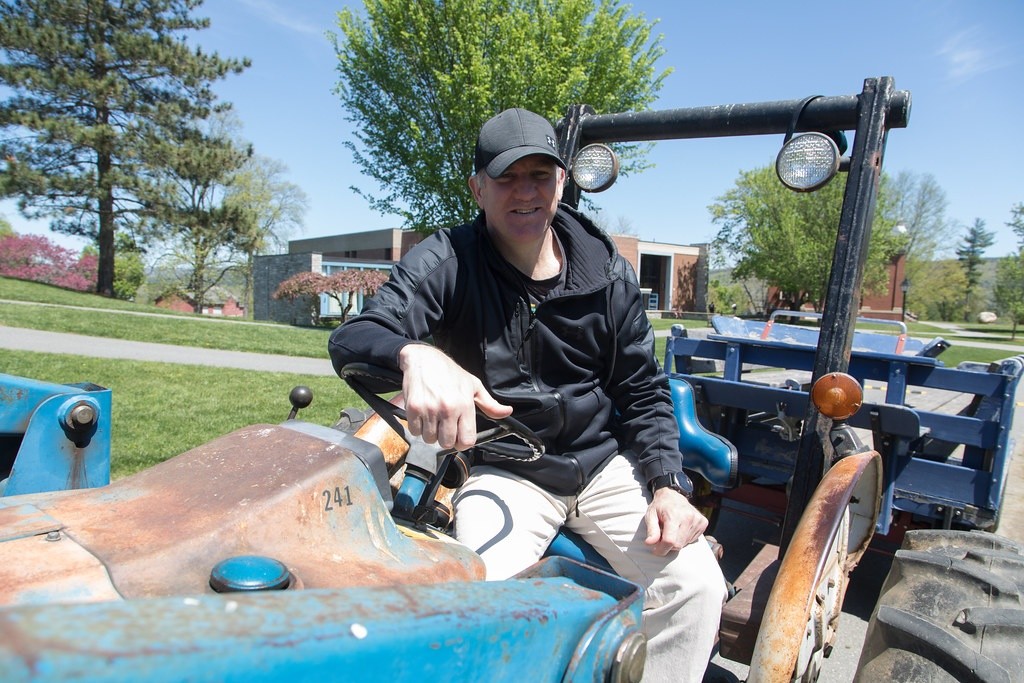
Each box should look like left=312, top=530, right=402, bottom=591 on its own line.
left=652, top=471, right=693, bottom=498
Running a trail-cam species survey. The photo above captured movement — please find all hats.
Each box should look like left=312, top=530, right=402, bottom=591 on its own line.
left=474, top=107, right=568, bottom=180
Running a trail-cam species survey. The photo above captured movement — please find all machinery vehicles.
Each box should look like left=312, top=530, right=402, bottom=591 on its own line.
left=0, top=78, right=1024, bottom=683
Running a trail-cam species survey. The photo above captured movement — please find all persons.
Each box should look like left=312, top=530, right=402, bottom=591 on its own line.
left=327, top=108, right=730, bottom=683
left=709, top=302, right=714, bottom=313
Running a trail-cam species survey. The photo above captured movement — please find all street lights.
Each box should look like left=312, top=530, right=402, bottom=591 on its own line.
left=901, top=279, right=908, bottom=325
